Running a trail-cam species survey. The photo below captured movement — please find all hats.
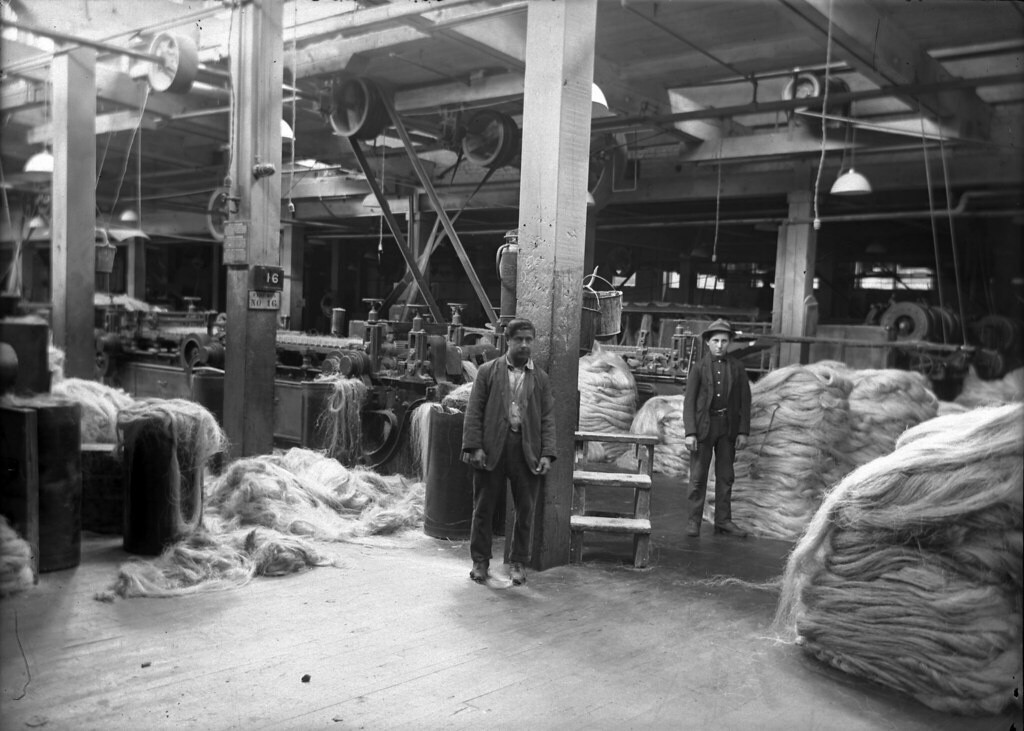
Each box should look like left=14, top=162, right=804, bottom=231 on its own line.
left=702, top=318, right=737, bottom=340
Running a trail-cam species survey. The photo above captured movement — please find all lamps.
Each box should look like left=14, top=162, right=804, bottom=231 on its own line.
left=24, top=68, right=54, bottom=173
left=362, top=140, right=380, bottom=207
left=830, top=100, right=881, bottom=196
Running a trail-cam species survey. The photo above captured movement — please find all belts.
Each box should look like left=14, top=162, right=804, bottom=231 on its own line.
left=711, top=409, right=727, bottom=416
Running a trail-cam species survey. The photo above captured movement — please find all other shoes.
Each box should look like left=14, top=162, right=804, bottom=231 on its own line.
left=510, top=561, right=525, bottom=584
left=471, top=559, right=488, bottom=581
left=686, top=517, right=701, bottom=536
left=715, top=520, right=747, bottom=537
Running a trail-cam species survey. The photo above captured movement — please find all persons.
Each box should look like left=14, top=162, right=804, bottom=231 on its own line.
left=683, top=319, right=752, bottom=539
left=461, top=319, right=557, bottom=586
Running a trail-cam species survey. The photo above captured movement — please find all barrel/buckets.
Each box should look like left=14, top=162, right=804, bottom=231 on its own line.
left=124, top=416, right=204, bottom=557
left=0, top=394, right=82, bottom=573
left=580, top=284, right=603, bottom=352
left=423, top=403, right=500, bottom=539
left=583, top=274, right=623, bottom=336
